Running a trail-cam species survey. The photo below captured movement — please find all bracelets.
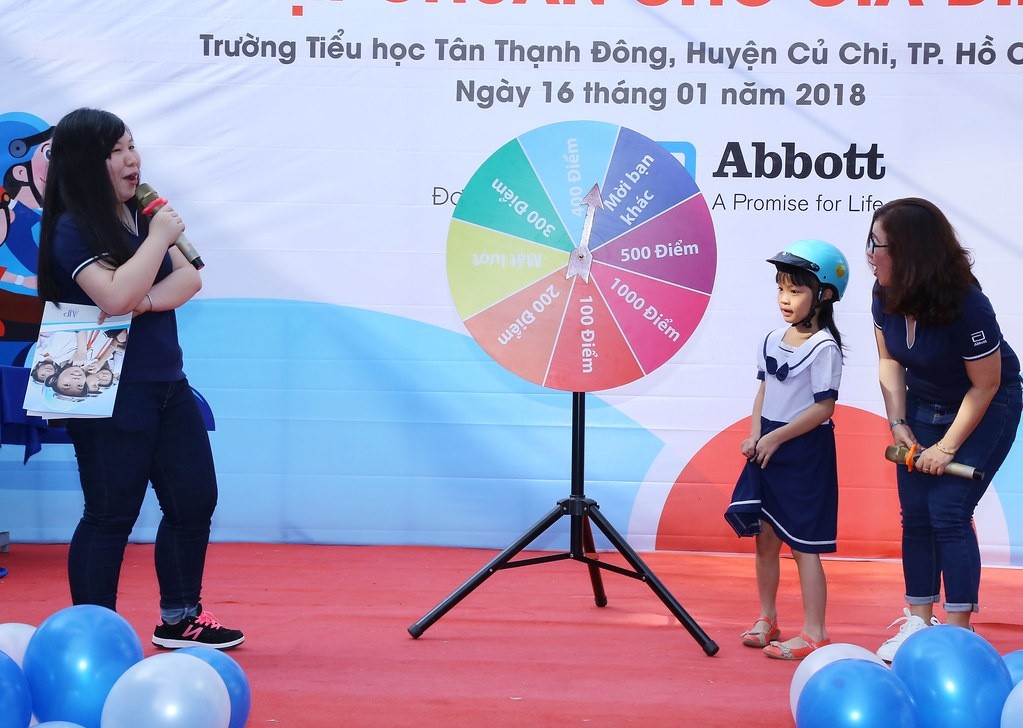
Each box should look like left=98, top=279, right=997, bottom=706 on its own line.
left=890, top=419, right=908, bottom=429
left=147, top=294, right=154, bottom=311
left=936, top=442, right=956, bottom=455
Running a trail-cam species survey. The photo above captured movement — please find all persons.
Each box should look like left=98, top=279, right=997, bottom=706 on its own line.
left=865, top=198, right=1023, bottom=662
left=724, top=240, right=849, bottom=659
left=31, top=328, right=129, bottom=402
left=39, top=108, right=244, bottom=651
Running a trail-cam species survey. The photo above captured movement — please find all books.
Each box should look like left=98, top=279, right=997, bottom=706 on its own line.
left=22, top=301, right=132, bottom=419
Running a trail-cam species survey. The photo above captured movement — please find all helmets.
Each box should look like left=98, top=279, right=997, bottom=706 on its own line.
left=765, top=239, right=850, bottom=302
left=32, top=361, right=111, bottom=413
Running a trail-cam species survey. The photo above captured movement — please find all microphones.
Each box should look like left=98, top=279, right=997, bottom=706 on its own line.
left=135, top=182, right=205, bottom=270
left=885, top=446, right=986, bottom=481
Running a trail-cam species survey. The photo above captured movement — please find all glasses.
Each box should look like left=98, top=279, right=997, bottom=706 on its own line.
left=870, top=238, right=888, bottom=254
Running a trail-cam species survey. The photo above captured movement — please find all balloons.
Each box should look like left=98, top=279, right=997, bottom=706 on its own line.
left=101, top=653, right=231, bottom=728
left=1000, top=650, right=1022, bottom=727
left=0, top=651, right=32, bottom=728
left=891, top=625, right=1013, bottom=728
left=0, top=622, right=39, bottom=727
left=173, top=646, right=251, bottom=728
left=797, top=659, right=920, bottom=728
left=22, top=605, right=144, bottom=728
left=790, top=643, right=890, bottom=725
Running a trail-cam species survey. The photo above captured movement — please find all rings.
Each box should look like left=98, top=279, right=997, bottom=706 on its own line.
left=923, top=467, right=929, bottom=472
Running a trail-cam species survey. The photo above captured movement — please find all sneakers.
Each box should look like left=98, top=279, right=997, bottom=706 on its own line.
left=151, top=603, right=245, bottom=649
left=740, top=616, right=780, bottom=647
left=763, top=633, right=831, bottom=659
left=875, top=607, right=930, bottom=662
